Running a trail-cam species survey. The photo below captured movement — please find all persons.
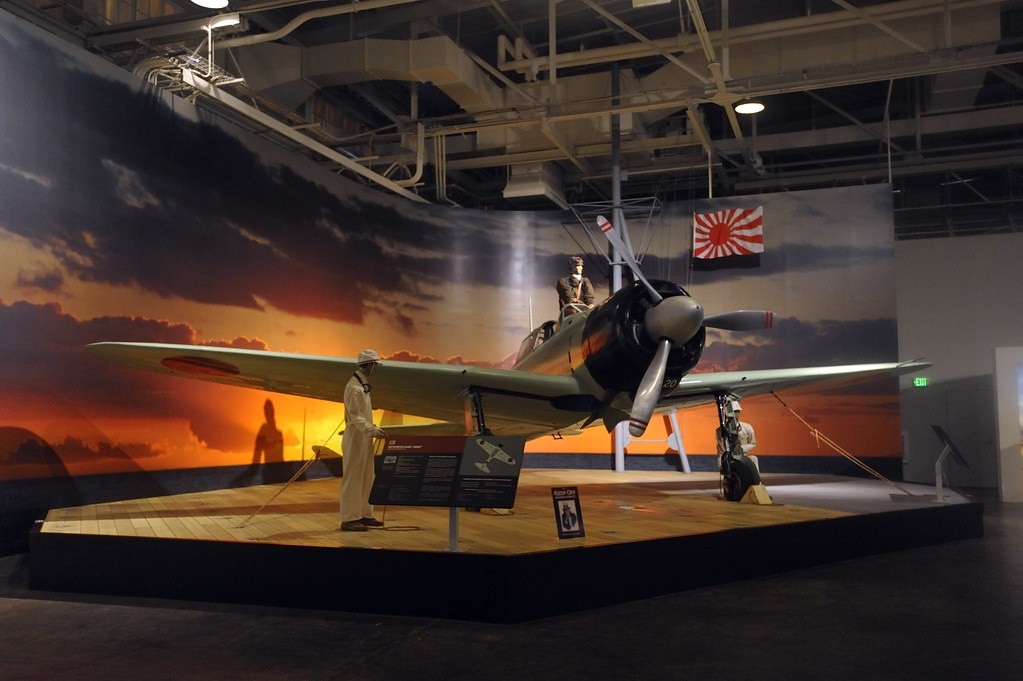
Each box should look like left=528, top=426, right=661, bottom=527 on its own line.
left=556, top=257, right=594, bottom=325
left=562, top=505, right=577, bottom=530
left=717, top=402, right=773, bottom=501
left=341, top=350, right=386, bottom=531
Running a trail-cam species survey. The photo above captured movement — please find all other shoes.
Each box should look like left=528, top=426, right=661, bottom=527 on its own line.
left=341, top=521, right=368, bottom=531
left=360, top=517, right=383, bottom=526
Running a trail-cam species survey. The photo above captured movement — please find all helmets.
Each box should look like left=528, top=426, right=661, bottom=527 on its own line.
left=725, top=400, right=743, bottom=415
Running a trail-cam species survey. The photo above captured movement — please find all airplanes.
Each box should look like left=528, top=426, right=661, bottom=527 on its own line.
left=80, top=212, right=937, bottom=514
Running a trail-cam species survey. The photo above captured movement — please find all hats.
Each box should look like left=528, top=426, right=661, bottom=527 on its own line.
left=567, top=257, right=583, bottom=274
left=562, top=505, right=571, bottom=510
left=357, top=350, right=383, bottom=366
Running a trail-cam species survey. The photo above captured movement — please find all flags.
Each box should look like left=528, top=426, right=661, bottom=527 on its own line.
left=694, top=206, right=764, bottom=259
